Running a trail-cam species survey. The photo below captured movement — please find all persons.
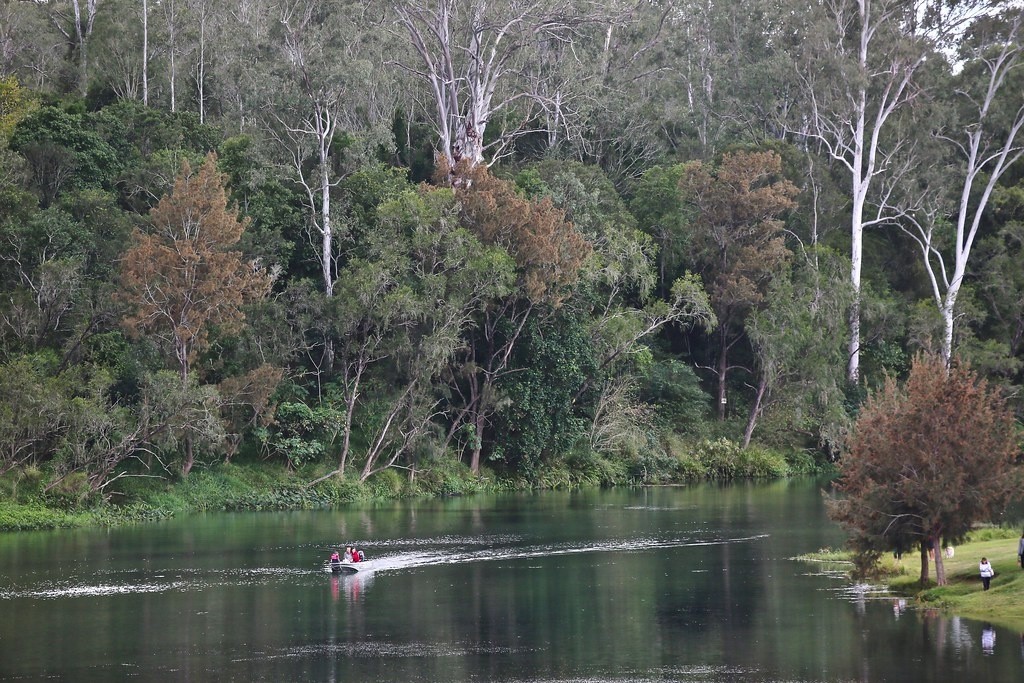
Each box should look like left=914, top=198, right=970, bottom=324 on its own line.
left=344, top=546, right=361, bottom=562
left=1018, top=534, right=1024, bottom=568
left=980, top=558, right=991, bottom=591
left=929, top=548, right=935, bottom=560
left=982, top=622, right=996, bottom=655
left=331, top=552, right=340, bottom=563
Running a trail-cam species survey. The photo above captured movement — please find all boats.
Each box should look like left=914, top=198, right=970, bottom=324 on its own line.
left=329, top=561, right=360, bottom=575
